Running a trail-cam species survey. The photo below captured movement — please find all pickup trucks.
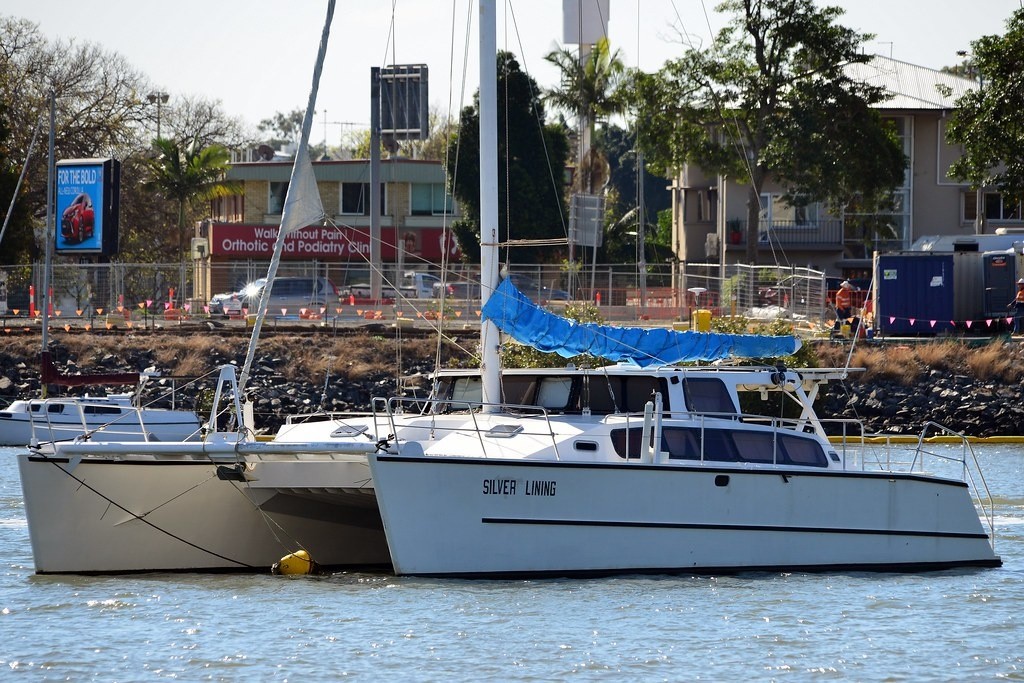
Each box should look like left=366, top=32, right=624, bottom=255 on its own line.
left=436, top=270, right=575, bottom=300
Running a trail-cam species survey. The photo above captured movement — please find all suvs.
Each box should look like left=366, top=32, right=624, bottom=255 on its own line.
left=208, top=274, right=343, bottom=318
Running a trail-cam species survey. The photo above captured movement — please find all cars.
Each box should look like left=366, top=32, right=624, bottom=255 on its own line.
left=759, top=275, right=861, bottom=308
left=62, top=195, right=96, bottom=243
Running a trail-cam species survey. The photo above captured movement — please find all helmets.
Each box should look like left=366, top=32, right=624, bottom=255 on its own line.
left=840, top=281, right=851, bottom=289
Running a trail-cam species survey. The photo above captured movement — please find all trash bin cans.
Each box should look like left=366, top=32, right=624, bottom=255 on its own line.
left=691, top=308, right=712, bottom=333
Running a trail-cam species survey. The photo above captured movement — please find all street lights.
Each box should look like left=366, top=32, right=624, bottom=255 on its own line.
left=956, top=51, right=985, bottom=234
left=148, top=92, right=168, bottom=138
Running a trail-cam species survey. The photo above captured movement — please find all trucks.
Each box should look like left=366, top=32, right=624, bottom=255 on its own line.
left=346, top=274, right=451, bottom=298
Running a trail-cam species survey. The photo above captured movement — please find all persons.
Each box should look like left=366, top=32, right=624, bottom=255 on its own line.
left=1006, top=277, right=1024, bottom=336
left=833, top=279, right=852, bottom=333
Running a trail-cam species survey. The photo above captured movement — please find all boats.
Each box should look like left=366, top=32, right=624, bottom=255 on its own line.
left=21, top=0, right=1001, bottom=577
left=0, top=393, right=201, bottom=448
